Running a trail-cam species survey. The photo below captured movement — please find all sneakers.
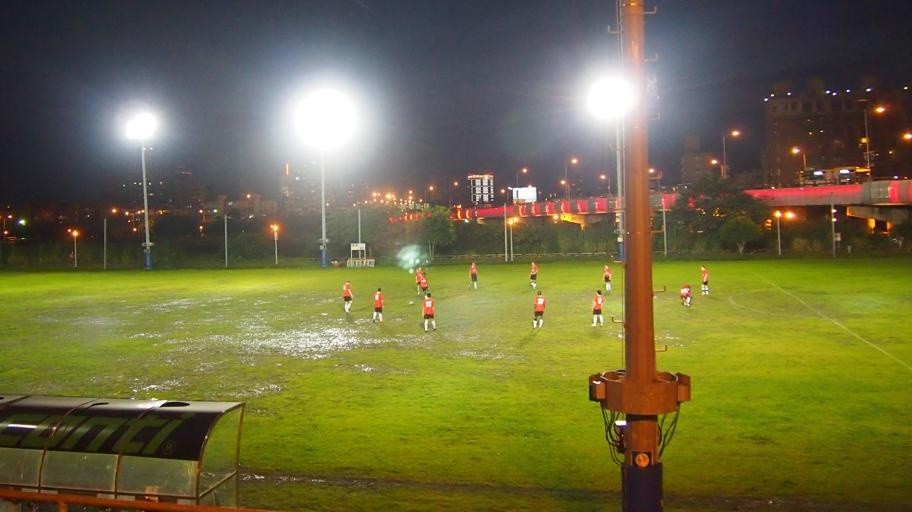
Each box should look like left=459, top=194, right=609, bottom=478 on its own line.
left=606, top=288, right=612, bottom=291
left=533, top=326, right=542, bottom=330
left=532, top=284, right=536, bottom=289
left=474, top=287, right=477, bottom=290
left=424, top=328, right=436, bottom=331
left=591, top=323, right=603, bottom=327
left=701, top=292, right=709, bottom=295
left=684, top=302, right=690, bottom=307
left=344, top=308, right=349, bottom=314
left=373, top=319, right=383, bottom=323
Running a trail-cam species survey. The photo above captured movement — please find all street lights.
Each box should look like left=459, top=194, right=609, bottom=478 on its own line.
left=773, top=211, right=782, bottom=255
left=125, top=109, right=164, bottom=266
left=708, top=106, right=886, bottom=183
left=269, top=223, right=281, bottom=265
left=498, top=188, right=517, bottom=263
left=584, top=77, right=642, bottom=262
left=295, top=81, right=345, bottom=267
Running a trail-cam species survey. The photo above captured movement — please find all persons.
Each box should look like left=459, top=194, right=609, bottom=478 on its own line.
left=529, top=260, right=538, bottom=290
left=415, top=265, right=425, bottom=292
left=532, top=290, right=548, bottom=330
left=419, top=273, right=430, bottom=294
left=420, top=292, right=438, bottom=332
left=342, top=281, right=353, bottom=314
left=467, top=260, right=479, bottom=289
left=603, top=264, right=612, bottom=291
left=701, top=264, right=710, bottom=297
left=592, top=289, right=605, bottom=327
left=371, top=286, right=384, bottom=324
left=680, top=283, right=691, bottom=307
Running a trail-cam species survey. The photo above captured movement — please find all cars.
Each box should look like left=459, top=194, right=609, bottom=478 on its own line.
left=0, top=209, right=146, bottom=269
